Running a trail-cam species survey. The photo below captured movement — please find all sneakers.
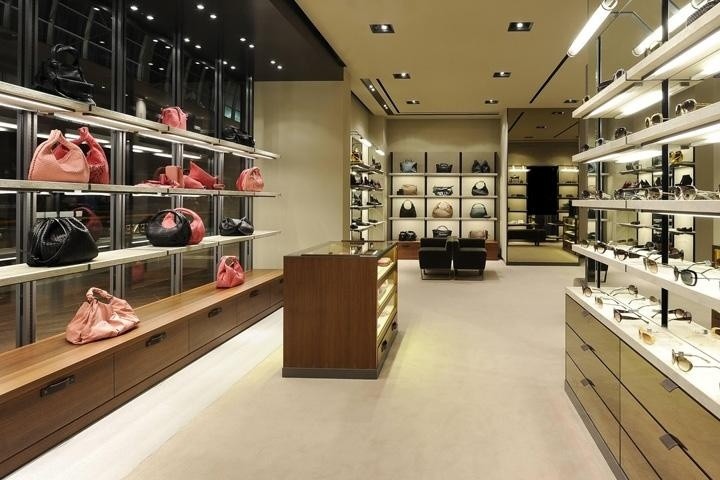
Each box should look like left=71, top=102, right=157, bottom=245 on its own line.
left=351, top=149, right=382, bottom=230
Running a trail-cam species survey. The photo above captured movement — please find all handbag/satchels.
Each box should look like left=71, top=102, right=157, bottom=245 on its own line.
left=219, top=216, right=254, bottom=235
left=67, top=287, right=141, bottom=345
left=27, top=217, right=98, bottom=267
left=28, top=127, right=109, bottom=184
left=397, top=157, right=490, bottom=241
left=146, top=208, right=205, bottom=247
left=73, top=207, right=103, bottom=242
left=155, top=106, right=188, bottom=130
left=221, top=125, right=255, bottom=147
left=217, top=256, right=244, bottom=288
left=235, top=167, right=265, bottom=192
left=27, top=43, right=93, bottom=102
left=144, top=160, right=225, bottom=189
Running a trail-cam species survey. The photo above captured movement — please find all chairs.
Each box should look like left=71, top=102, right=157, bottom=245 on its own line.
left=417, top=236, right=488, bottom=280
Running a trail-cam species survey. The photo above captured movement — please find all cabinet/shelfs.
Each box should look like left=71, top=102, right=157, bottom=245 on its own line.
left=282, top=240, right=398, bottom=380
left=564, top=0, right=720, bottom=480
left=0, top=80, right=284, bottom=480
left=344, top=127, right=501, bottom=260
left=558, top=165, right=579, bottom=254
left=508, top=164, right=528, bottom=229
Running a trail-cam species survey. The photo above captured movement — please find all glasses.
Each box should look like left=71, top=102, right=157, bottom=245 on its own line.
left=612, top=70, right=624, bottom=82
left=696, top=327, right=720, bottom=341
left=581, top=185, right=720, bottom=202
left=595, top=138, right=608, bottom=147
left=643, top=257, right=673, bottom=274
left=611, top=238, right=634, bottom=246
left=651, top=309, right=692, bottom=324
left=583, top=144, right=594, bottom=151
left=688, top=259, right=720, bottom=276
left=629, top=241, right=662, bottom=252
left=582, top=94, right=590, bottom=103
left=671, top=349, right=720, bottom=372
left=594, top=240, right=615, bottom=254
left=644, top=113, right=671, bottom=128
left=610, top=285, right=638, bottom=296
left=615, top=127, right=632, bottom=140
left=639, top=328, right=673, bottom=345
left=673, top=265, right=720, bottom=288
left=648, top=248, right=685, bottom=261
left=595, top=296, right=627, bottom=310
left=628, top=296, right=661, bottom=309
left=675, top=99, right=712, bottom=117
left=614, top=248, right=646, bottom=261
left=582, top=284, right=610, bottom=297
left=613, top=308, right=650, bottom=325
left=582, top=240, right=589, bottom=247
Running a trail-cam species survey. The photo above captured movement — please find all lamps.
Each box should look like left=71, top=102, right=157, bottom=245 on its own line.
left=566, top=0, right=617, bottom=58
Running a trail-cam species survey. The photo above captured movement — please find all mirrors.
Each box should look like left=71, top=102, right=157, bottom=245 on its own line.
left=506, top=107, right=580, bottom=266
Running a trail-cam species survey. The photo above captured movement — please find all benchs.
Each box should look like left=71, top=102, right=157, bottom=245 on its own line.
left=507, top=229, right=546, bottom=246
